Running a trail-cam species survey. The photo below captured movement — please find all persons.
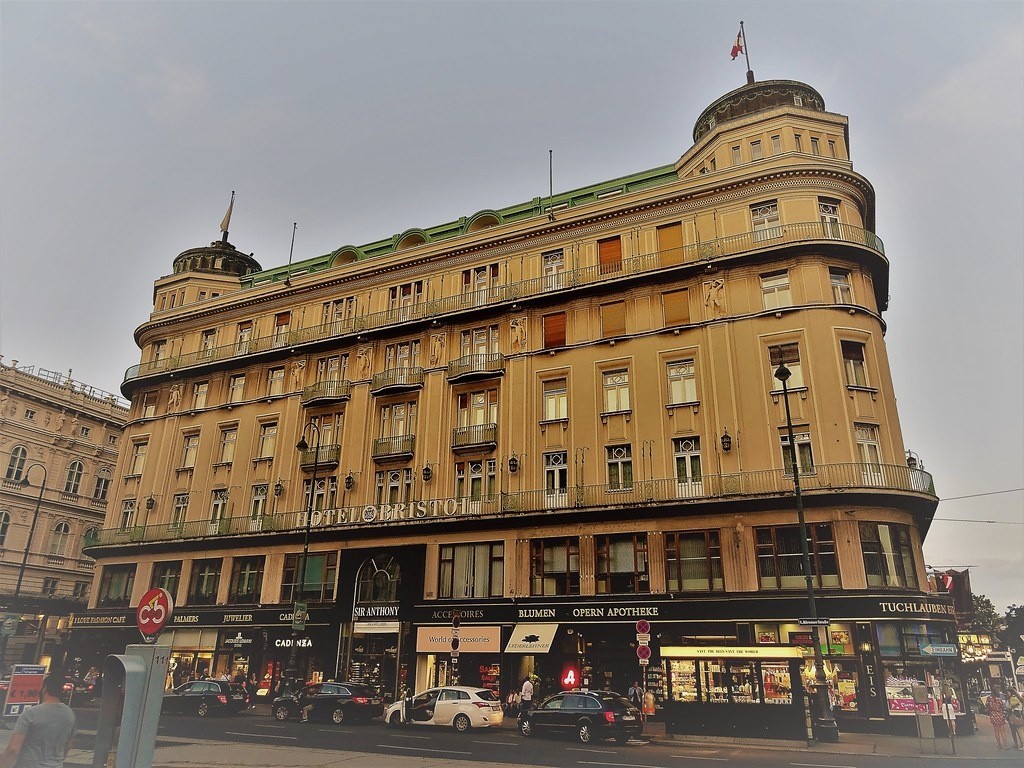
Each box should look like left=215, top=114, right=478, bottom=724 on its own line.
left=83, top=666, right=100, bottom=702
left=70, top=414, right=79, bottom=435
left=164, top=663, right=299, bottom=709
left=56, top=410, right=66, bottom=432
left=432, top=335, right=445, bottom=367
left=0, top=391, right=9, bottom=416
left=937, top=684, right=956, bottom=738
left=293, top=360, right=305, bottom=391
left=356, top=351, right=369, bottom=381
left=510, top=318, right=525, bottom=354
left=627, top=680, right=644, bottom=723
left=731, top=675, right=741, bottom=690
left=411, top=692, right=439, bottom=722
left=984, top=686, right=1024, bottom=750
left=503, top=676, right=534, bottom=717
left=0, top=675, right=77, bottom=768
left=704, top=279, right=724, bottom=319
left=167, top=385, right=180, bottom=412
left=298, top=688, right=316, bottom=722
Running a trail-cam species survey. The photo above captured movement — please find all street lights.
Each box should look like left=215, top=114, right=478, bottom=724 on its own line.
left=283, top=422, right=320, bottom=680
left=774, top=363, right=840, bottom=742
left=10, top=463, right=49, bottom=612
left=961, top=640, right=992, bottom=691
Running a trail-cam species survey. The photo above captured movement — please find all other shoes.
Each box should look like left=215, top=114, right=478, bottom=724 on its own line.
left=300, top=719, right=308, bottom=722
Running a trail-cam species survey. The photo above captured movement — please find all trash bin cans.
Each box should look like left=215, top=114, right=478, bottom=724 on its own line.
left=916, top=712, right=935, bottom=738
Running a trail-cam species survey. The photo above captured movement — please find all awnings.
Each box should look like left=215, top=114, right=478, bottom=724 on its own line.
left=504, top=624, right=560, bottom=654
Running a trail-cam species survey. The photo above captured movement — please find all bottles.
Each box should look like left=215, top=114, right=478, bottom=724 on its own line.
left=647, top=666, right=664, bottom=710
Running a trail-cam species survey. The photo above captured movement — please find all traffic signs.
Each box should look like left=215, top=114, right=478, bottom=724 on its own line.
left=919, top=644, right=957, bottom=657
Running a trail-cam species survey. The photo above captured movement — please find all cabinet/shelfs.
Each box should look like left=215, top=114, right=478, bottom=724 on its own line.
left=487, top=664, right=500, bottom=685
left=668, top=670, right=788, bottom=704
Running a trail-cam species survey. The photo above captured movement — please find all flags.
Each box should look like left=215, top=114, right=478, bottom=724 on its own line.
left=942, top=573, right=953, bottom=589
left=220, top=195, right=235, bottom=232
left=730, top=25, right=746, bottom=61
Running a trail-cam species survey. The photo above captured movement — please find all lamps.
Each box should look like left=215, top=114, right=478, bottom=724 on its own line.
left=905, top=449, right=924, bottom=474
left=721, top=426, right=732, bottom=451
left=144, top=492, right=163, bottom=508
left=423, top=460, right=440, bottom=482
left=508, top=450, right=528, bottom=474
left=958, top=636, right=975, bottom=656
left=345, top=469, right=363, bottom=491
left=275, top=477, right=294, bottom=496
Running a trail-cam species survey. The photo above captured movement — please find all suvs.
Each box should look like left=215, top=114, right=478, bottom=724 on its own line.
left=516, top=690, right=644, bottom=747
left=978, top=691, right=1013, bottom=715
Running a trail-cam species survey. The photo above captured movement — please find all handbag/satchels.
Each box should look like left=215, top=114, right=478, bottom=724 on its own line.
left=952, top=701, right=960, bottom=713
left=1009, top=712, right=1023, bottom=725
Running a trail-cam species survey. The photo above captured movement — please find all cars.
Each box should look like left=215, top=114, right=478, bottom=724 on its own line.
left=384, top=686, right=504, bottom=734
left=0, top=673, right=94, bottom=709
left=271, top=682, right=384, bottom=724
left=159, top=680, right=250, bottom=719
